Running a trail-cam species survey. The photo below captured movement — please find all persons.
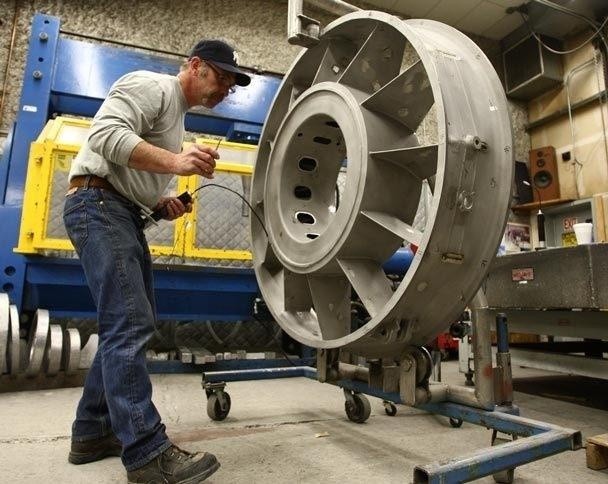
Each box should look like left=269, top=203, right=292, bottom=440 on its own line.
left=63, top=37, right=252, bottom=483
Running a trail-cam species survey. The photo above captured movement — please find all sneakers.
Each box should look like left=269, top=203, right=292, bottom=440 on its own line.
left=126, top=444, right=220, bottom=484
left=68, top=431, right=122, bottom=464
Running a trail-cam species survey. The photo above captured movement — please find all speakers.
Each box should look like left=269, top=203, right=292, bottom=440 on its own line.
left=528, top=146, right=561, bottom=201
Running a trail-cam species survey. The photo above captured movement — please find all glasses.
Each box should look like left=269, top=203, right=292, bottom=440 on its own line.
left=201, top=60, right=236, bottom=94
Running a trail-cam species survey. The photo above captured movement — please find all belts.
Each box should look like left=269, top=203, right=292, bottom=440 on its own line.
left=68, top=175, right=114, bottom=191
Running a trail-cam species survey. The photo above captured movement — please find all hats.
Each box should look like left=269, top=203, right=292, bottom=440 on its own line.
left=190, top=39, right=251, bottom=86
